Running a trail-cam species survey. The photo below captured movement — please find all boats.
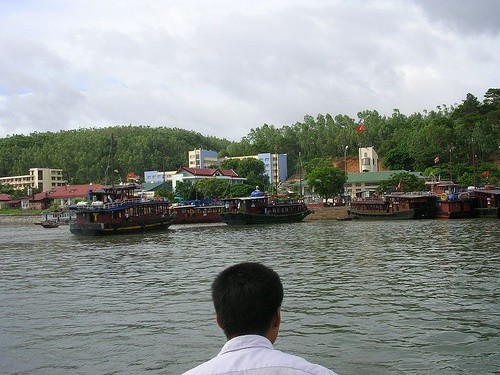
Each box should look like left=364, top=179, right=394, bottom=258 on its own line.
left=168, top=171, right=225, bottom=225
left=66, top=131, right=178, bottom=236
left=219, top=143, right=315, bottom=226
left=346, top=138, right=500, bottom=221
left=335, top=216, right=353, bottom=221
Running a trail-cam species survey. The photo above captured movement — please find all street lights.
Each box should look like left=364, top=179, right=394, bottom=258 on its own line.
left=345, top=145, right=349, bottom=207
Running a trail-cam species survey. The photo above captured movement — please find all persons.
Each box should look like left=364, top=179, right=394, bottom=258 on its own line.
left=180, top=262, right=338, bottom=375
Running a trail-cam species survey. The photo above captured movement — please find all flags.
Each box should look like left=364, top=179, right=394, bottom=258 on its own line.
left=482, top=171, right=488, bottom=176
left=396, top=179, right=402, bottom=190
left=355, top=124, right=364, bottom=132
left=127, top=172, right=140, bottom=179
left=434, top=157, right=439, bottom=164
